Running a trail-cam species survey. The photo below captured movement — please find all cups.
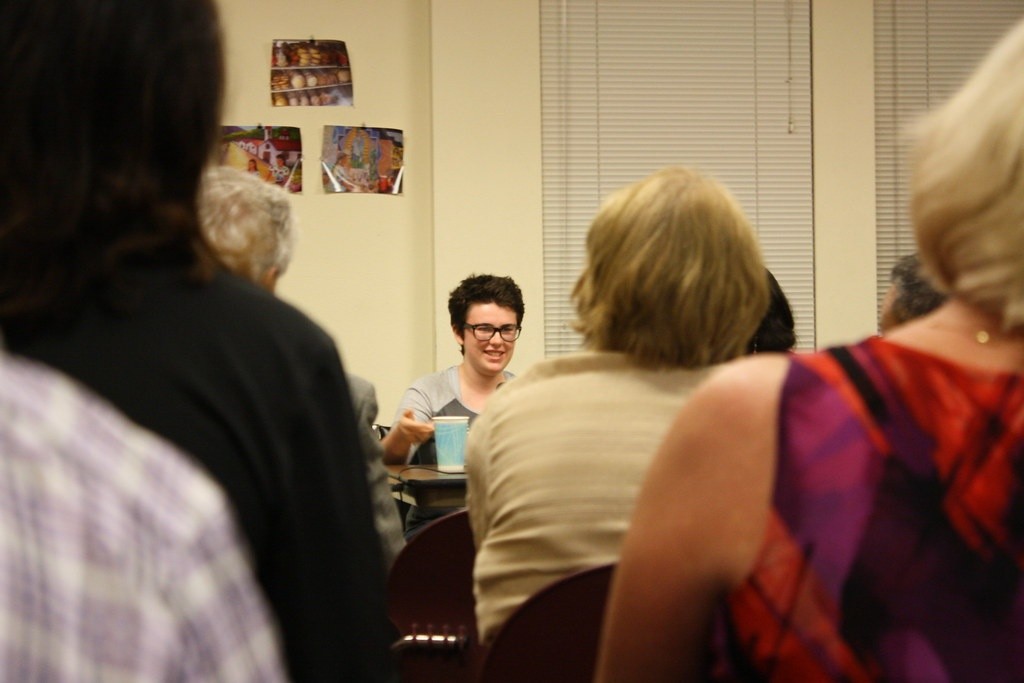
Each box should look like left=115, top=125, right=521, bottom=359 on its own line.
left=431, top=415, right=470, bottom=472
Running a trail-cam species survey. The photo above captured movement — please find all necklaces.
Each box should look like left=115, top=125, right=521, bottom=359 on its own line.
left=932, top=320, right=1001, bottom=342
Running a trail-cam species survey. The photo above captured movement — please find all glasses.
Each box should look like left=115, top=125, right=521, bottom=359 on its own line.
left=459, top=321, right=523, bottom=342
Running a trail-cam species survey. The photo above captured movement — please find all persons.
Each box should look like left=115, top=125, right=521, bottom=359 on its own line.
left=463, top=172, right=770, bottom=648
left=744, top=269, right=797, bottom=354
left=1, top=352, right=285, bottom=683
left=381, top=276, right=526, bottom=539
left=881, top=252, right=946, bottom=334
left=200, top=167, right=406, bottom=567
left=599, top=25, right=1024, bottom=683
left=0, top=0, right=391, bottom=683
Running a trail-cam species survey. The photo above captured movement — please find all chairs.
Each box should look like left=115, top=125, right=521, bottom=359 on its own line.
left=476, top=564, right=615, bottom=683
left=383, top=511, right=475, bottom=683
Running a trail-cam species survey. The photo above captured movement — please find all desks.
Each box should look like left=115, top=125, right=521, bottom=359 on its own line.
left=383, top=463, right=466, bottom=512
